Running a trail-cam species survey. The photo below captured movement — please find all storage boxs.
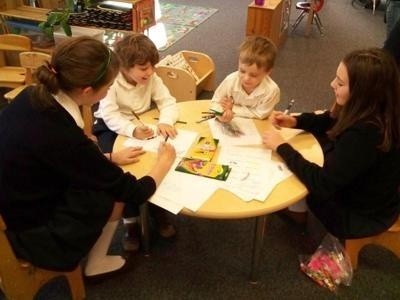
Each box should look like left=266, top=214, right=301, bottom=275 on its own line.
left=53, top=26, right=105, bottom=48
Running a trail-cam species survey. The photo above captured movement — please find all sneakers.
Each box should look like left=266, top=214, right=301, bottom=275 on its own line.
left=277, top=207, right=309, bottom=225
left=121, top=215, right=141, bottom=250
left=145, top=220, right=176, bottom=239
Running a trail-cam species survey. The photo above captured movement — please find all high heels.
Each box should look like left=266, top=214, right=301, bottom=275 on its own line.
left=83, top=253, right=141, bottom=286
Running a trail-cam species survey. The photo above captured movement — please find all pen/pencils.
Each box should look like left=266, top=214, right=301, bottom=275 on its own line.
left=165, top=135, right=170, bottom=142
left=196, top=108, right=222, bottom=123
left=284, top=98, right=295, bottom=115
left=154, top=118, right=186, bottom=124
left=131, top=111, right=145, bottom=126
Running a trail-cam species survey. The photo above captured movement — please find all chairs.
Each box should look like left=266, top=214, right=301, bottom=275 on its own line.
left=0, top=34, right=33, bottom=88
left=4, top=52, right=52, bottom=107
left=344, top=217, right=400, bottom=273
left=292, top=0, right=326, bottom=35
left=0, top=215, right=87, bottom=300
left=82, top=102, right=97, bottom=142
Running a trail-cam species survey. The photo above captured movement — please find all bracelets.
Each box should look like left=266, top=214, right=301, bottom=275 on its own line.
left=110, top=154, right=113, bottom=162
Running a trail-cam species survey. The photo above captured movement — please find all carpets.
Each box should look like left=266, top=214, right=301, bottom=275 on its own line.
left=157, top=0, right=220, bottom=52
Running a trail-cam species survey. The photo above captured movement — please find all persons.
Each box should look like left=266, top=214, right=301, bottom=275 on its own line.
left=262, top=49, right=400, bottom=239
left=94, top=33, right=178, bottom=256
left=211, top=35, right=280, bottom=123
left=0, top=35, right=176, bottom=279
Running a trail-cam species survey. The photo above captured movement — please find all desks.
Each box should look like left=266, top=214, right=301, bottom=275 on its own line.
left=247, top=0, right=291, bottom=48
left=112, top=98, right=325, bottom=282
left=0, top=5, right=52, bottom=45
